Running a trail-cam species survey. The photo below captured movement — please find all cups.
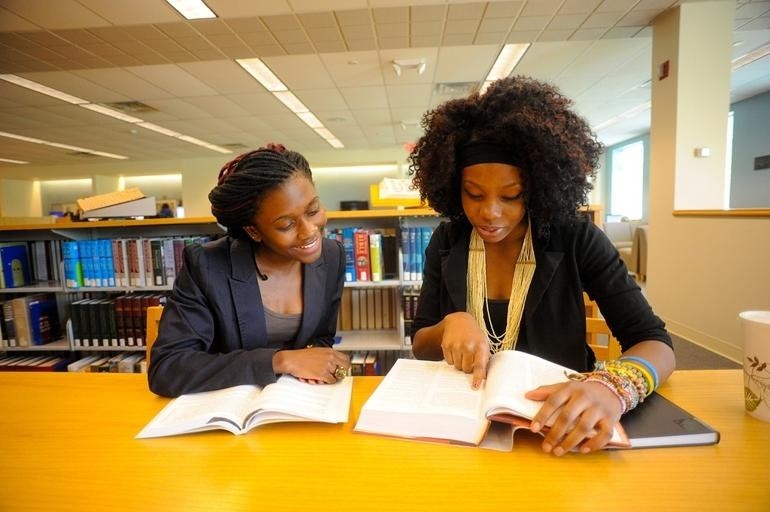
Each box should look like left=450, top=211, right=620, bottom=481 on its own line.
left=738, top=307, right=770, bottom=423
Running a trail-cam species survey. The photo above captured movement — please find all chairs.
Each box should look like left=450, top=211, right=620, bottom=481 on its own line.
left=583, top=315, right=623, bottom=362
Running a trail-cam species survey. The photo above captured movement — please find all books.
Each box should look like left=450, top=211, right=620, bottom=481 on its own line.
left=353, top=350, right=632, bottom=451
left=0, top=232, right=226, bottom=373
left=351, top=350, right=400, bottom=375
left=323, top=226, right=433, bottom=345
left=607, top=390, right=720, bottom=449
left=135, top=373, right=352, bottom=438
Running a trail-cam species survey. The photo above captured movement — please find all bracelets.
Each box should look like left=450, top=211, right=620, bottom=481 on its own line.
left=564, top=352, right=658, bottom=415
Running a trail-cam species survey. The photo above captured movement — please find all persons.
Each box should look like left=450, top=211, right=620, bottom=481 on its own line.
left=147, top=146, right=352, bottom=397
left=408, top=76, right=677, bottom=457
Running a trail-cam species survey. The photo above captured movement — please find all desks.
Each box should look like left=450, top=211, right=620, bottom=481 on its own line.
left=0, top=365, right=769, bottom=512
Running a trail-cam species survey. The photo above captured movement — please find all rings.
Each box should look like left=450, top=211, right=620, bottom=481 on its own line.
left=334, top=365, right=347, bottom=379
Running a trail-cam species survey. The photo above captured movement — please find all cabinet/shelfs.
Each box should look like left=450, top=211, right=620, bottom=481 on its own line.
left=0, top=203, right=605, bottom=351
